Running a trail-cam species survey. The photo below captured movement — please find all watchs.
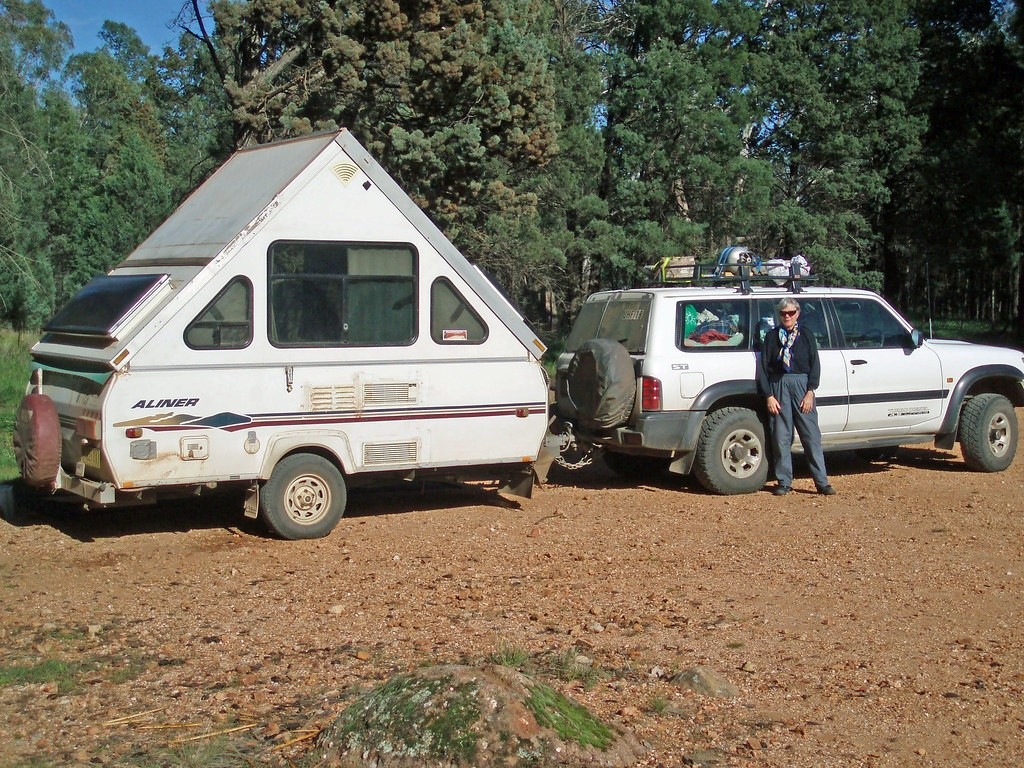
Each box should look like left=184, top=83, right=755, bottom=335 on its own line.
left=807, top=389, right=816, bottom=393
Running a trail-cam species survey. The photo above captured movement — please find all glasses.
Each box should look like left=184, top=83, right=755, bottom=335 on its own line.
left=779, top=310, right=798, bottom=317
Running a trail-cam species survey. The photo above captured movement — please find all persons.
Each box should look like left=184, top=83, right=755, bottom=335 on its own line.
left=759, top=298, right=836, bottom=495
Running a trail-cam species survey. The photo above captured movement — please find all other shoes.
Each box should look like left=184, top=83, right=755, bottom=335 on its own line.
left=775, top=483, right=790, bottom=495
left=817, top=486, right=835, bottom=495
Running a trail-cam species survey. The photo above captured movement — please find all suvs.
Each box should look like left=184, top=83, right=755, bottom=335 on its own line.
left=551, top=245, right=1024, bottom=495
